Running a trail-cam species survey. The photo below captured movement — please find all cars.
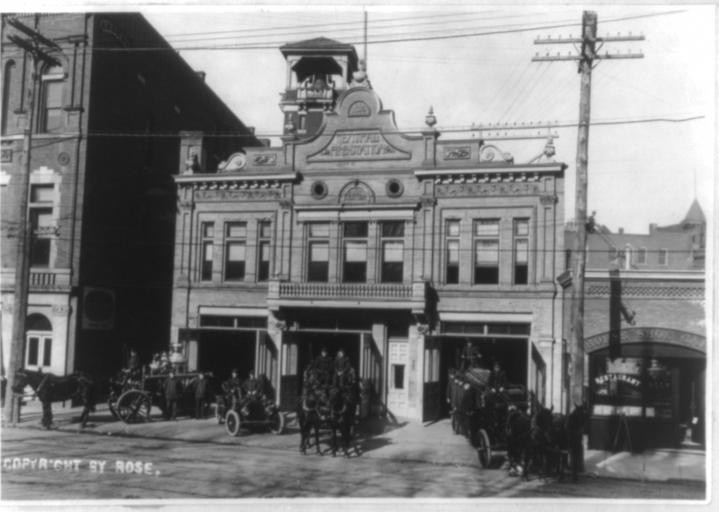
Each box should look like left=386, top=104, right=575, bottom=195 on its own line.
left=214, top=387, right=286, bottom=438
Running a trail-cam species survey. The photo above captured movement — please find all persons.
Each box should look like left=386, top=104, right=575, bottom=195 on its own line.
left=244, top=370, right=262, bottom=400
left=333, top=348, right=352, bottom=376
left=315, top=345, right=334, bottom=376
left=223, top=368, right=243, bottom=400
left=162, top=367, right=186, bottom=422
left=191, top=372, right=211, bottom=420
left=489, top=358, right=509, bottom=393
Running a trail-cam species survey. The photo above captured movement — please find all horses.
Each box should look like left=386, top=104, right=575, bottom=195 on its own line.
left=296, top=384, right=360, bottom=459
left=503, top=399, right=590, bottom=483
left=11, top=367, right=96, bottom=429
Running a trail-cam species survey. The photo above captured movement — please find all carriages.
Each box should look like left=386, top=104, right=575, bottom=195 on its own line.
left=10, top=363, right=213, bottom=430
left=296, top=364, right=364, bottom=458
left=443, top=365, right=589, bottom=484
